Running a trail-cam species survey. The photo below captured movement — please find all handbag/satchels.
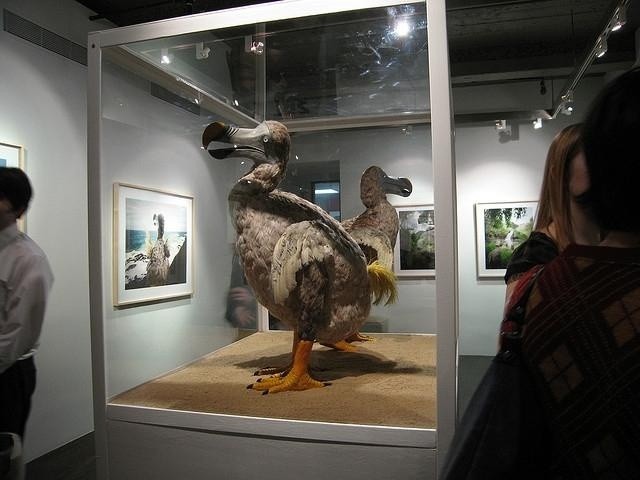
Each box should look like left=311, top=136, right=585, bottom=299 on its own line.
left=438, top=265, right=546, bottom=479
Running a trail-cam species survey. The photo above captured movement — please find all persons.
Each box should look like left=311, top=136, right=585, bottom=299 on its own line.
left=497, top=63, right=638, bottom=478
left=221, top=253, right=292, bottom=333
left=497, top=119, right=607, bottom=316
left=0, top=166, right=52, bottom=453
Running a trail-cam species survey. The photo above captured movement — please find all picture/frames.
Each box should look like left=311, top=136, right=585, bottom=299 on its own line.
left=391, top=199, right=541, bottom=280
left=112, top=180, right=195, bottom=309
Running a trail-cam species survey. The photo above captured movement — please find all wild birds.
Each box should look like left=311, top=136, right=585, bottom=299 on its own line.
left=319, top=166, right=413, bottom=353
left=144, top=213, right=170, bottom=286
left=203, top=121, right=398, bottom=397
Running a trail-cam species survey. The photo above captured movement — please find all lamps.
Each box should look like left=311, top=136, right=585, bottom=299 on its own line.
left=491, top=15, right=629, bottom=132
